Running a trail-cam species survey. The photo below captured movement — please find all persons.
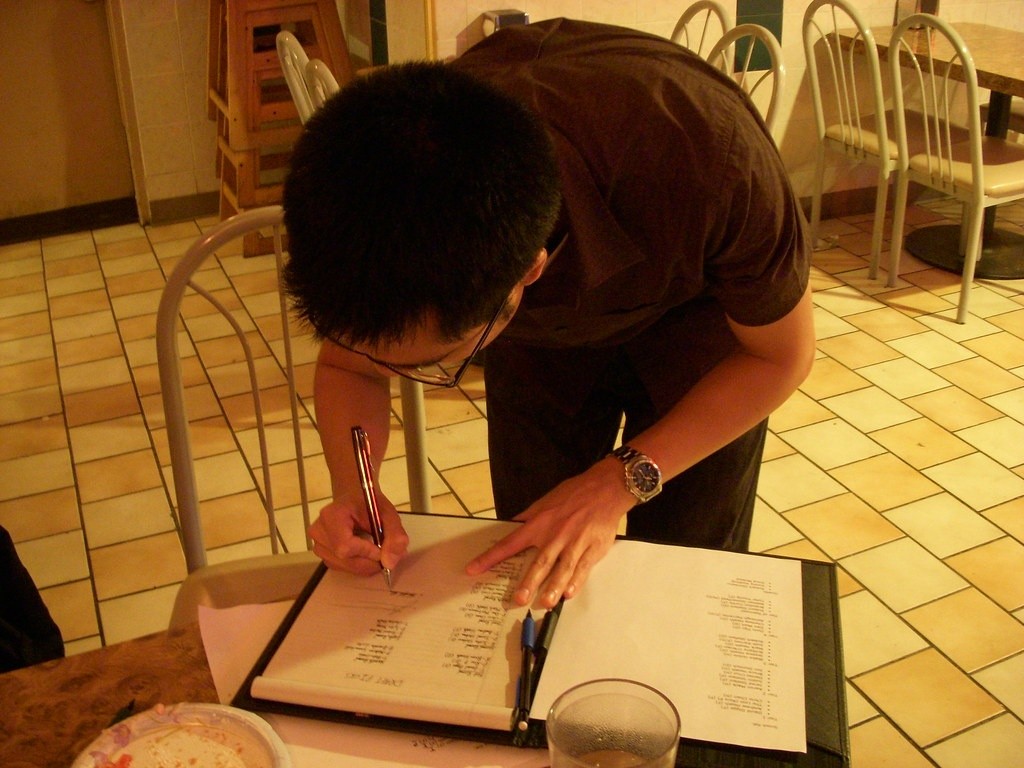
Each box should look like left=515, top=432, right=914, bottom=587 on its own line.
left=281, top=16, right=816, bottom=607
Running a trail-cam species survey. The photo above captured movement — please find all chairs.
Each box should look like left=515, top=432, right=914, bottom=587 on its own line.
left=704, top=23, right=789, bottom=137
left=671, top=0, right=733, bottom=79
left=275, top=30, right=323, bottom=124
left=887, top=11, right=1024, bottom=325
left=304, top=59, right=340, bottom=110
left=801, top=0, right=970, bottom=280
left=157, top=208, right=430, bottom=629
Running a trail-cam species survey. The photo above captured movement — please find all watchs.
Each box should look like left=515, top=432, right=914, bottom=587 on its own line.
left=605, top=445, right=663, bottom=503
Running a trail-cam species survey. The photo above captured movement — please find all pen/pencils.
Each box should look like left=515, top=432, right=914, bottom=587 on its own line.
left=514, top=608, right=535, bottom=731
left=510, top=593, right=566, bottom=737
left=350, top=425, right=393, bottom=591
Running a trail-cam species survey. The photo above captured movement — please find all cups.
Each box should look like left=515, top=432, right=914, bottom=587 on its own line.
left=546, top=678, right=680, bottom=768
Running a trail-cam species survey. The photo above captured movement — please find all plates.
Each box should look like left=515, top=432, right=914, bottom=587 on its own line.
left=71, top=702, right=291, bottom=768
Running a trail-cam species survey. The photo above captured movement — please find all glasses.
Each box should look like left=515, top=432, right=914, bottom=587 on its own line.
left=309, top=285, right=516, bottom=388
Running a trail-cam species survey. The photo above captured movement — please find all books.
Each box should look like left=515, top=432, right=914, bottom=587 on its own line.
left=248, top=512, right=547, bottom=731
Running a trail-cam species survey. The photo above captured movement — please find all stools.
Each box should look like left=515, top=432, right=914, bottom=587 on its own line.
left=205, top=0, right=353, bottom=259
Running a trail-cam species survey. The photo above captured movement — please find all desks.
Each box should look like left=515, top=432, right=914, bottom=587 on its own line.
left=0, top=594, right=644, bottom=768
left=830, top=21, right=1024, bottom=281
